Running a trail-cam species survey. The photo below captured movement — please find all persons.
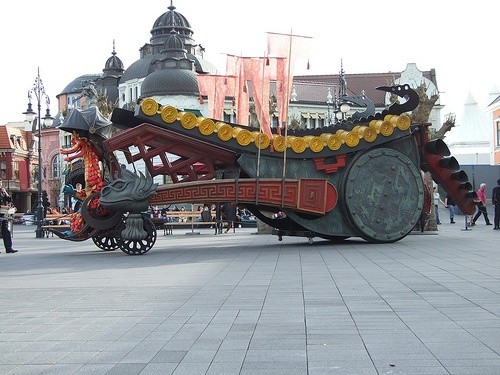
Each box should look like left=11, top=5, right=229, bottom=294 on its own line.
left=161, top=204, right=217, bottom=223
left=492, top=178, right=500, bottom=230
left=71, top=182, right=82, bottom=211
left=470, top=182, right=492, bottom=226
left=0, top=178, right=18, bottom=253
left=433, top=187, right=445, bottom=225
left=444, top=191, right=456, bottom=223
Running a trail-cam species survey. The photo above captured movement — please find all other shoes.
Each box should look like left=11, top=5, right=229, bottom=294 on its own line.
left=486, top=223, right=493, bottom=225
left=210, top=226, right=212, bottom=229
left=471, top=224, right=474, bottom=226
left=6, top=249, right=18, bottom=253
left=437, top=223, right=442, bottom=224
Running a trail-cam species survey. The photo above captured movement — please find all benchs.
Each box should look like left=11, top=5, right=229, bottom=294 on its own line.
left=41, top=214, right=72, bottom=238
left=163, top=211, right=218, bottom=235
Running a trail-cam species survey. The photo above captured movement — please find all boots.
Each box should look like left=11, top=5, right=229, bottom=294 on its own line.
left=451, top=218, right=455, bottom=223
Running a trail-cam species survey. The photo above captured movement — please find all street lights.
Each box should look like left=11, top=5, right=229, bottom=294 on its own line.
left=332, top=58, right=350, bottom=125
left=20, top=65, right=55, bottom=238
